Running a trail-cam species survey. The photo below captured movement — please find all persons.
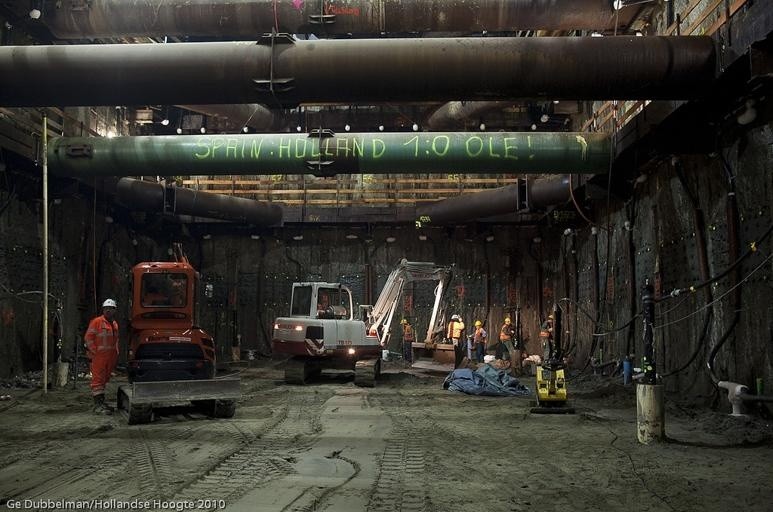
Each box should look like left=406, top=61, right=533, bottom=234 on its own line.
left=446, top=314, right=465, bottom=369
left=539, top=314, right=559, bottom=365
left=83, top=298, right=121, bottom=416
left=498, top=317, right=515, bottom=363
left=317, top=300, right=331, bottom=317
left=465, top=320, right=486, bottom=364
left=399, top=318, right=415, bottom=363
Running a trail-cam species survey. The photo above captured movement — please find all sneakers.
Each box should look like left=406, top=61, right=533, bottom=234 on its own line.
left=92, top=392, right=115, bottom=416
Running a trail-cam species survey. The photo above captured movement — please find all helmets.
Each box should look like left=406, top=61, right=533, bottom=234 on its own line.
left=450, top=315, right=459, bottom=319
left=102, top=298, right=118, bottom=308
left=474, top=321, right=482, bottom=327
left=399, top=319, right=407, bottom=325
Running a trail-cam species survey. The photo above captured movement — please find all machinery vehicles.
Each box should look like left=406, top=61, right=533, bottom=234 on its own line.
left=271, top=257, right=473, bottom=388
left=117, top=241, right=241, bottom=425
